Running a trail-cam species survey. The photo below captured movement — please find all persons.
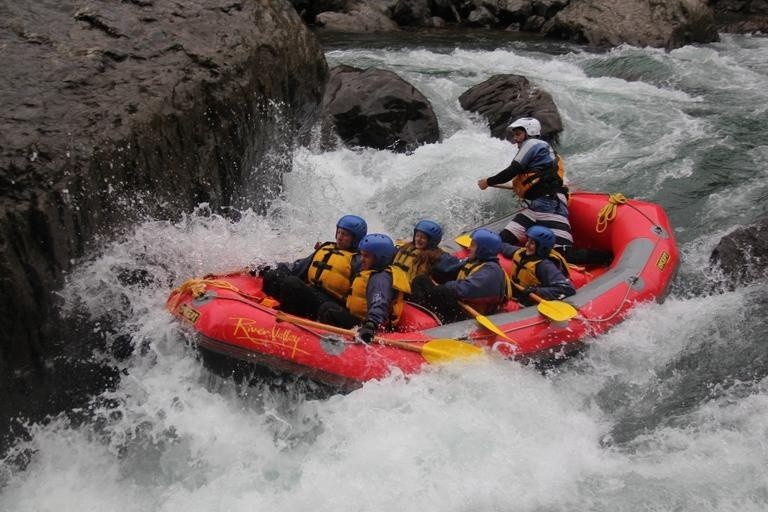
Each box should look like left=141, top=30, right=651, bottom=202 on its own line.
left=411, top=229, right=506, bottom=320
left=314, top=235, right=397, bottom=343
left=477, top=117, right=613, bottom=267
left=264, top=215, right=367, bottom=305
left=393, top=221, right=458, bottom=297
left=502, top=226, right=578, bottom=298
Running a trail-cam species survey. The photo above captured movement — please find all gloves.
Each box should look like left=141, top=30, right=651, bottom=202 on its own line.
left=523, top=286, right=536, bottom=297
left=357, top=321, right=375, bottom=344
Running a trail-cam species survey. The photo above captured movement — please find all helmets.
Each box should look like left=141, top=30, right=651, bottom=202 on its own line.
left=526, top=226, right=555, bottom=259
left=472, top=231, right=501, bottom=258
left=413, top=220, right=442, bottom=248
left=508, top=117, right=542, bottom=137
left=335, top=215, right=367, bottom=247
left=358, top=233, right=394, bottom=268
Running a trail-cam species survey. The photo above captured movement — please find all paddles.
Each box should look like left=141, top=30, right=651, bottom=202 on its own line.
left=279, top=313, right=483, bottom=364
left=510, top=277, right=578, bottom=323
left=430, top=275, right=521, bottom=345
